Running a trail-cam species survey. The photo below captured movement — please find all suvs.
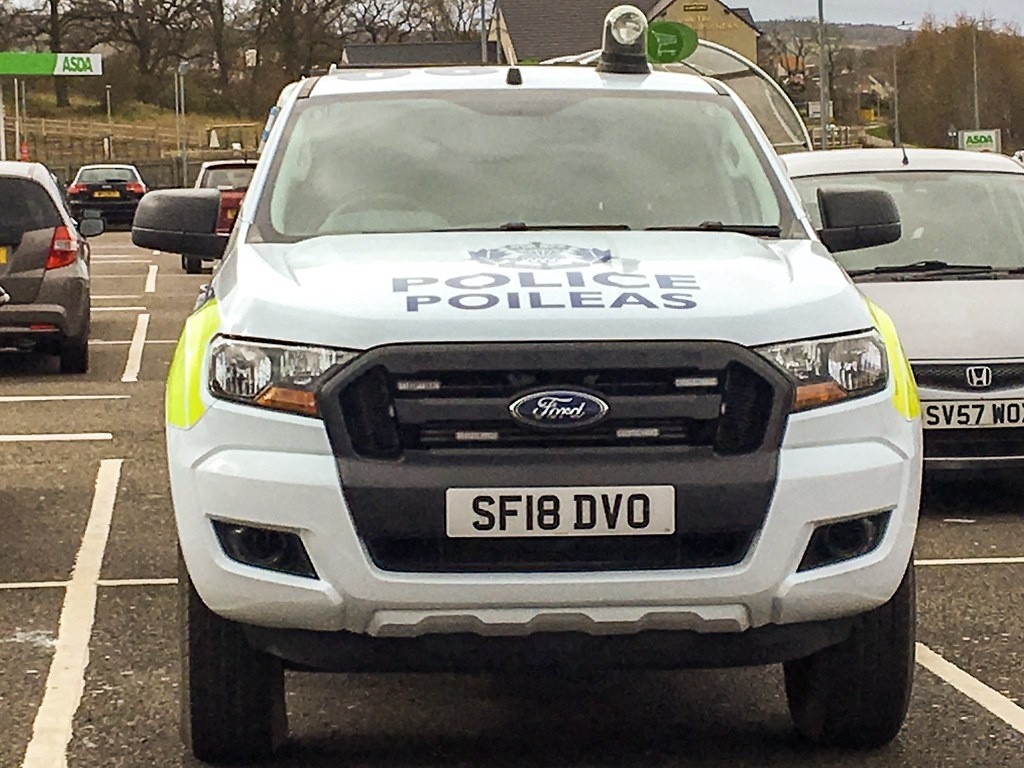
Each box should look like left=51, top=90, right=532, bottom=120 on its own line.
left=129, top=3, right=927, bottom=759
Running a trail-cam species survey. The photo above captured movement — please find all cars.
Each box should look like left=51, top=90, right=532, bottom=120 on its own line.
left=180, top=157, right=258, bottom=274
left=63, top=163, right=149, bottom=229
left=0, top=160, right=106, bottom=372
left=771, top=145, right=1024, bottom=496
